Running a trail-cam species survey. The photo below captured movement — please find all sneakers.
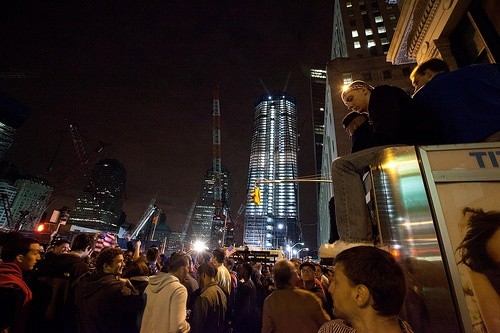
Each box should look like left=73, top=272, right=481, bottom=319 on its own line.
left=318, top=239, right=376, bottom=259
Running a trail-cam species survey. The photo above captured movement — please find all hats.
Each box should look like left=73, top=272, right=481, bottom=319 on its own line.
left=342, top=111, right=360, bottom=131
left=299, top=262, right=316, bottom=271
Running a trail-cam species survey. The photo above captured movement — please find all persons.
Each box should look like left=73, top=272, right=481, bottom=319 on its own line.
left=0, top=231, right=337, bottom=333
left=318, top=80, right=423, bottom=255
left=454, top=207, right=500, bottom=273
left=409, top=58, right=451, bottom=100
left=317, top=246, right=413, bottom=333
